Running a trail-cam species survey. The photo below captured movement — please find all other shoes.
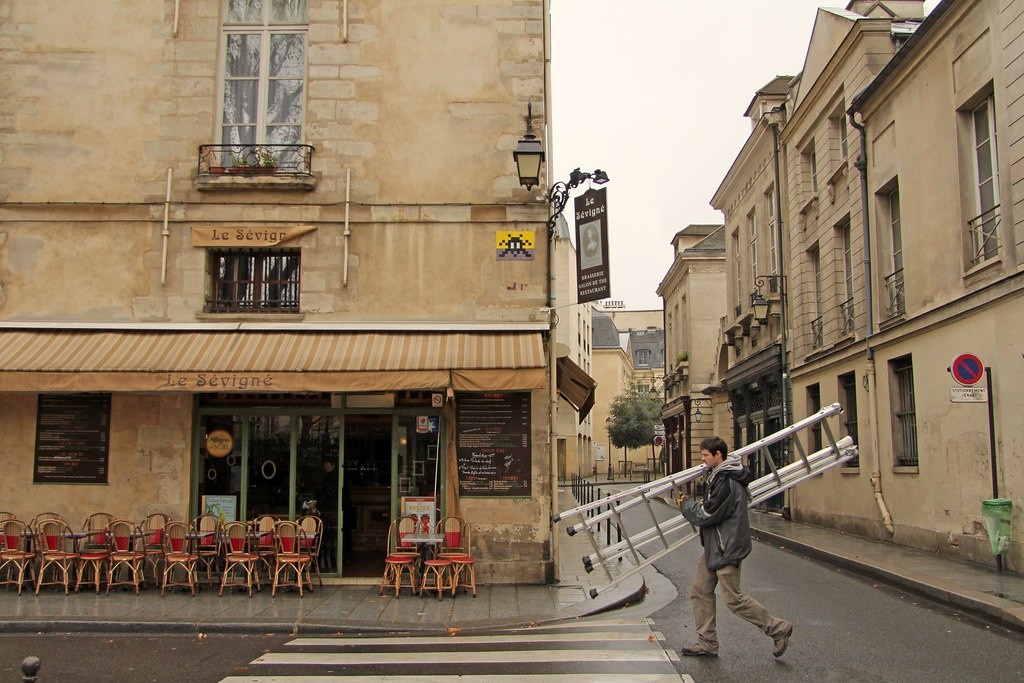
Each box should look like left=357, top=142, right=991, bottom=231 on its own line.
left=772, top=623, right=795, bottom=658
left=682, top=643, right=718, bottom=657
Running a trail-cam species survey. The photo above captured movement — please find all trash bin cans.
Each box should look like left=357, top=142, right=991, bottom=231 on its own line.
left=983, top=499, right=1012, bottom=554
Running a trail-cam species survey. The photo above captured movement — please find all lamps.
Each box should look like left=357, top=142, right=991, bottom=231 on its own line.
left=751, top=276, right=788, bottom=325
left=513, top=103, right=545, bottom=191
left=695, top=409, right=702, bottom=423
left=547, top=168, right=610, bottom=242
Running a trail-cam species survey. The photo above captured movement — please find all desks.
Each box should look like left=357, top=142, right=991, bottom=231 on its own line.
left=273, top=534, right=321, bottom=593
left=0, top=530, right=100, bottom=588
left=401, top=534, right=445, bottom=595
left=166, top=532, right=216, bottom=592
left=222, top=532, right=269, bottom=592
left=104, top=532, right=151, bottom=590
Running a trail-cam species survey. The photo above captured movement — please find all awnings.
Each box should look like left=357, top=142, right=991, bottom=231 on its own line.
left=557, top=342, right=597, bottom=425
left=0, top=321, right=551, bottom=394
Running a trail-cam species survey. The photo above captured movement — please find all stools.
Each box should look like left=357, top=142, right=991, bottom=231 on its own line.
left=419, top=560, right=454, bottom=602
left=449, top=558, right=477, bottom=598
left=380, top=558, right=417, bottom=598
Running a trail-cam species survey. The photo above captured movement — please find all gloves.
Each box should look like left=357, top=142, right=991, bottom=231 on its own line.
left=698, top=498, right=705, bottom=506
left=675, top=491, right=686, bottom=508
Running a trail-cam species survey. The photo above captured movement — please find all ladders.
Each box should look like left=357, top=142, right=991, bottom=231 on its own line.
left=553, top=401, right=860, bottom=599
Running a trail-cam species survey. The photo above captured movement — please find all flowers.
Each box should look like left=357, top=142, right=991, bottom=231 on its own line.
left=231, top=152, right=278, bottom=168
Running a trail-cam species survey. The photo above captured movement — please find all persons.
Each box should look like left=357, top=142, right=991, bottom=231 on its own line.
left=583, top=226, right=599, bottom=261
left=676, top=436, right=793, bottom=658
left=315, top=457, right=352, bottom=566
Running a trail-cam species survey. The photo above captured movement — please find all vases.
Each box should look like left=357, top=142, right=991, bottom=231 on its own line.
left=228, top=168, right=278, bottom=174
left=209, top=167, right=226, bottom=173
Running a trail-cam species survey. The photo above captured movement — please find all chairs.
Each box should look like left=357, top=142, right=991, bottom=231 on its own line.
left=0, top=512, right=324, bottom=597
left=382, top=516, right=477, bottom=601
